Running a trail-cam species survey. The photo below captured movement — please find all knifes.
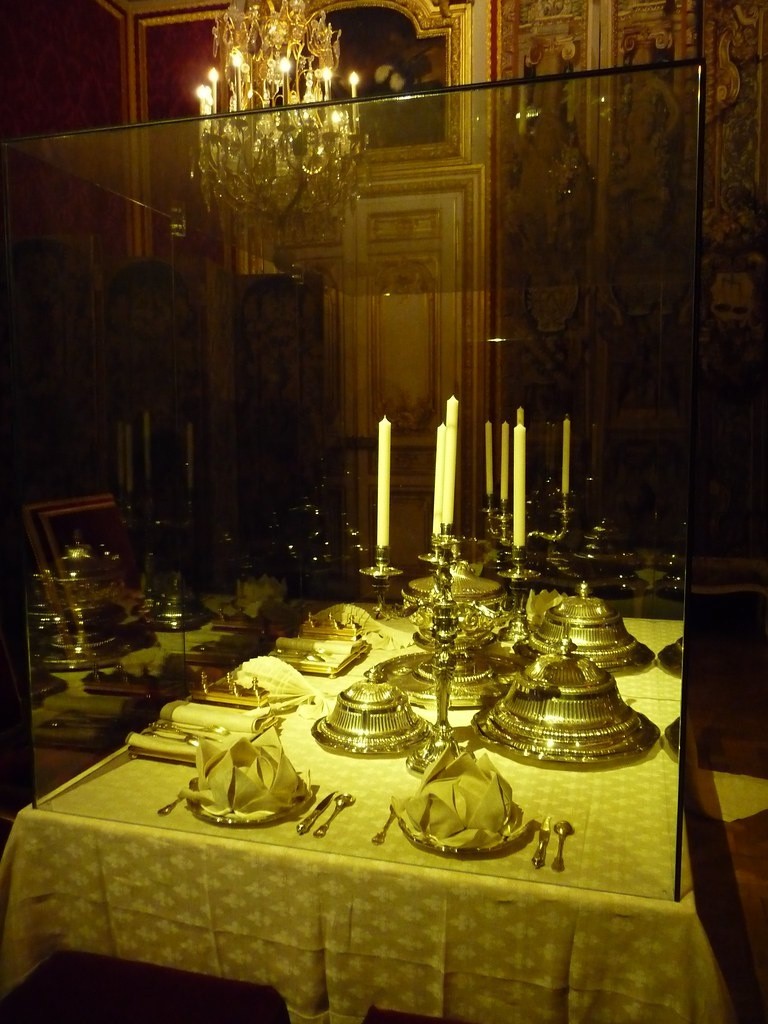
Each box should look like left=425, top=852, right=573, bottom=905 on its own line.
left=296, top=791, right=337, bottom=835
left=152, top=725, right=223, bottom=743
left=531, top=816, right=550, bottom=868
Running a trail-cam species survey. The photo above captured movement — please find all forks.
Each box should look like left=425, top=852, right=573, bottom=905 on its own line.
left=163, top=720, right=231, bottom=736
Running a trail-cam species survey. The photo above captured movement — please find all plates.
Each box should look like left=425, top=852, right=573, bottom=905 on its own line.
left=470, top=704, right=661, bottom=762
left=513, top=636, right=655, bottom=674
left=310, top=716, right=435, bottom=753
left=186, top=782, right=313, bottom=825
left=397, top=801, right=530, bottom=854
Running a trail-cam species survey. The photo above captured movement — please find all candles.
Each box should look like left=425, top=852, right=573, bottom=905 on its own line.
left=499, top=420, right=512, bottom=504
left=183, top=420, right=194, bottom=488
left=123, top=421, right=134, bottom=492
left=141, top=408, right=152, bottom=482
left=431, top=396, right=459, bottom=534
left=559, top=416, right=573, bottom=500
left=116, top=420, right=126, bottom=482
left=482, top=419, right=494, bottom=495
left=510, top=426, right=527, bottom=548
left=373, top=414, right=395, bottom=549
left=513, top=400, right=529, bottom=426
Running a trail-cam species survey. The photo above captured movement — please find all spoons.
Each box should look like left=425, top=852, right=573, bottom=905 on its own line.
left=142, top=731, right=199, bottom=747
left=313, top=794, right=357, bottom=838
left=157, top=777, right=197, bottom=819
left=551, top=821, right=574, bottom=873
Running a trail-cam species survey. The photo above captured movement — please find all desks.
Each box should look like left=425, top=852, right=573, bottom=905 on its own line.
left=1, top=598, right=768, bottom=1023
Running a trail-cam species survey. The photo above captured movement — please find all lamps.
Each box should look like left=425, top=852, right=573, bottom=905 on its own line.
left=190, top=1, right=361, bottom=224
